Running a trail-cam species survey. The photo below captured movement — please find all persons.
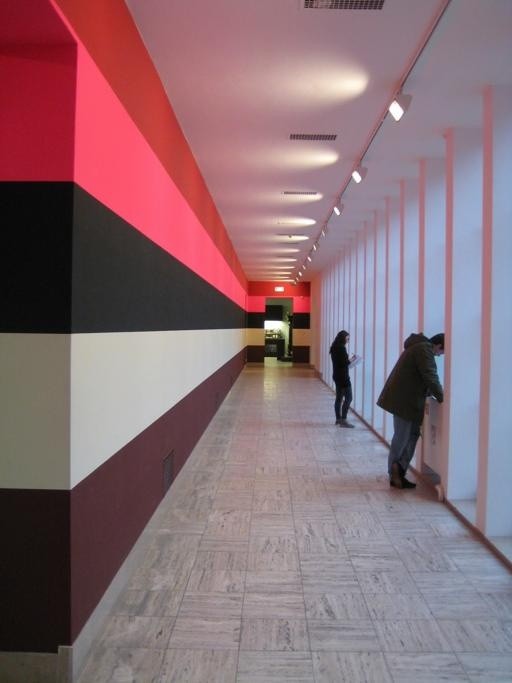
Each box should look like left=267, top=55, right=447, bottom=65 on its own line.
left=329, top=331, right=356, bottom=428
left=376, top=333, right=444, bottom=488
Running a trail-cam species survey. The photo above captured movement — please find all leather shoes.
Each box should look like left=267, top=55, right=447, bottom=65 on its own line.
left=335, top=418, right=355, bottom=428
left=389, top=462, right=417, bottom=489
